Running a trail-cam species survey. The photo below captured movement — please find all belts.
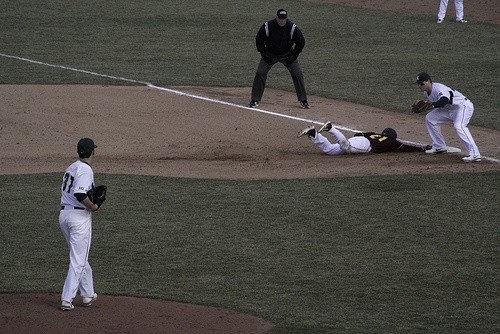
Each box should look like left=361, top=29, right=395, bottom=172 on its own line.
left=61, top=206, right=85, bottom=210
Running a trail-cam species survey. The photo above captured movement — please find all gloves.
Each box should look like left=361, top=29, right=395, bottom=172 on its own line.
left=285, top=53, right=297, bottom=64
left=261, top=50, right=272, bottom=64
left=410, top=100, right=430, bottom=114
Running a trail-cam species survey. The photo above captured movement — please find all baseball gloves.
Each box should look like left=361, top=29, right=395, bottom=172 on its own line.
left=410, top=99, right=427, bottom=113
left=88, top=185, right=108, bottom=211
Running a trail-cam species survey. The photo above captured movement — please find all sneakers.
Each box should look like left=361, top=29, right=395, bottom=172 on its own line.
left=299, top=101, right=310, bottom=109
left=83, top=292, right=98, bottom=307
left=248, top=100, right=258, bottom=107
left=425, top=144, right=447, bottom=154
left=61, top=301, right=74, bottom=310
left=297, top=125, right=316, bottom=138
left=462, top=153, right=481, bottom=162
left=317, top=119, right=332, bottom=133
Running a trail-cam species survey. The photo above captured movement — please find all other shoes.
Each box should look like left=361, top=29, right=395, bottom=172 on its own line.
left=437, top=19, right=443, bottom=24
left=459, top=19, right=467, bottom=23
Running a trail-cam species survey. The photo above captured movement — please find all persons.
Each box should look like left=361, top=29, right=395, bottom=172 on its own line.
left=437, top=0, right=467, bottom=23
left=249, top=9, right=310, bottom=109
left=59, top=138, right=107, bottom=311
left=410, top=73, right=481, bottom=161
left=299, top=120, right=432, bottom=154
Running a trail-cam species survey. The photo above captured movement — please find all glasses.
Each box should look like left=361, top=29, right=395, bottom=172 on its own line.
left=418, top=83, right=425, bottom=86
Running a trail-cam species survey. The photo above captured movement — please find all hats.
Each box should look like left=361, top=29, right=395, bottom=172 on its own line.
left=77, top=138, right=97, bottom=154
left=277, top=9, right=288, bottom=19
left=415, top=72, right=429, bottom=82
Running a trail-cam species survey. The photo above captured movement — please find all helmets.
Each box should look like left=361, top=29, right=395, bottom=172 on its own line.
left=382, top=128, right=397, bottom=138
